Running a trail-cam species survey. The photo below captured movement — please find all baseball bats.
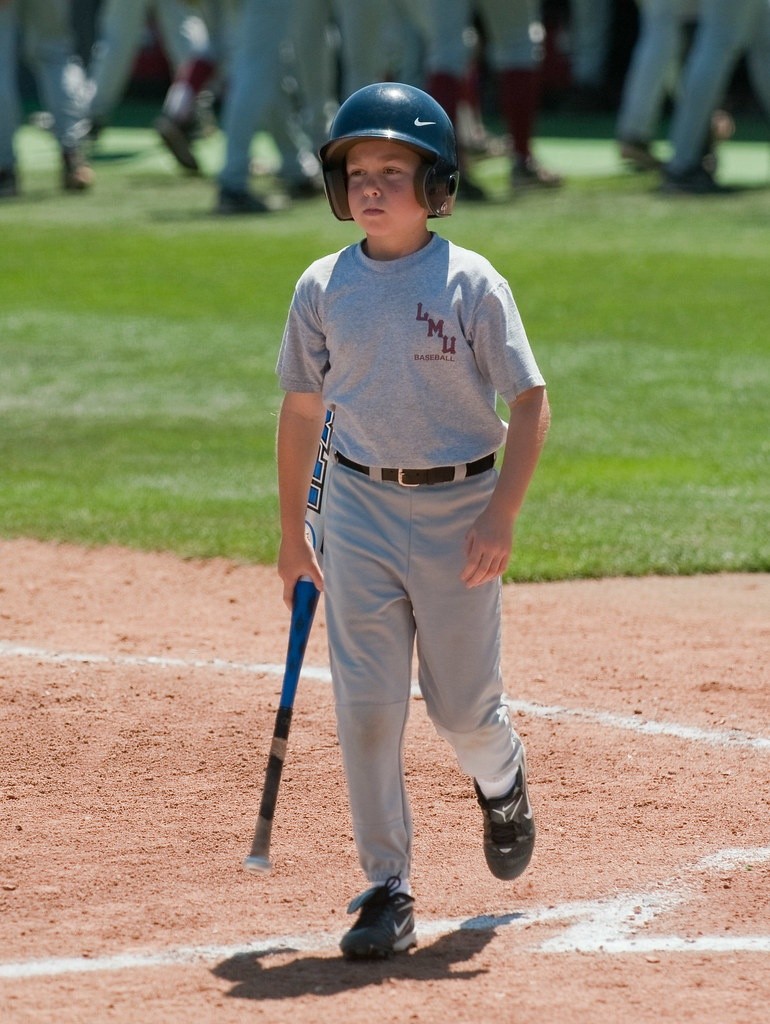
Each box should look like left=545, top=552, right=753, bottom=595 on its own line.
left=243, top=404, right=332, bottom=876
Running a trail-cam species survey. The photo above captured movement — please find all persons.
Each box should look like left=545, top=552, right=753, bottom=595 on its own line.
left=278, top=80, right=550, bottom=962
left=0, top=0, right=770, bottom=216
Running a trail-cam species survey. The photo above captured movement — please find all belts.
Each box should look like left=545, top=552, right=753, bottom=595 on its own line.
left=333, top=452, right=494, bottom=487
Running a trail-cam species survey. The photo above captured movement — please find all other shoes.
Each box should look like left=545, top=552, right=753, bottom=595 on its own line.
left=661, top=167, right=725, bottom=197
left=510, top=160, right=565, bottom=190
left=158, top=115, right=199, bottom=168
left=619, top=142, right=661, bottom=170
left=61, top=145, right=94, bottom=187
left=218, top=188, right=271, bottom=215
left=285, top=177, right=326, bottom=201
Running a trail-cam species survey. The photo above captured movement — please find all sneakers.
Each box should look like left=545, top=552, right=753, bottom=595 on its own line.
left=473, top=729, right=536, bottom=882
left=340, top=870, right=418, bottom=960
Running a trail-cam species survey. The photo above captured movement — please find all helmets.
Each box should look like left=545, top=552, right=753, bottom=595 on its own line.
left=318, top=82, right=459, bottom=220
